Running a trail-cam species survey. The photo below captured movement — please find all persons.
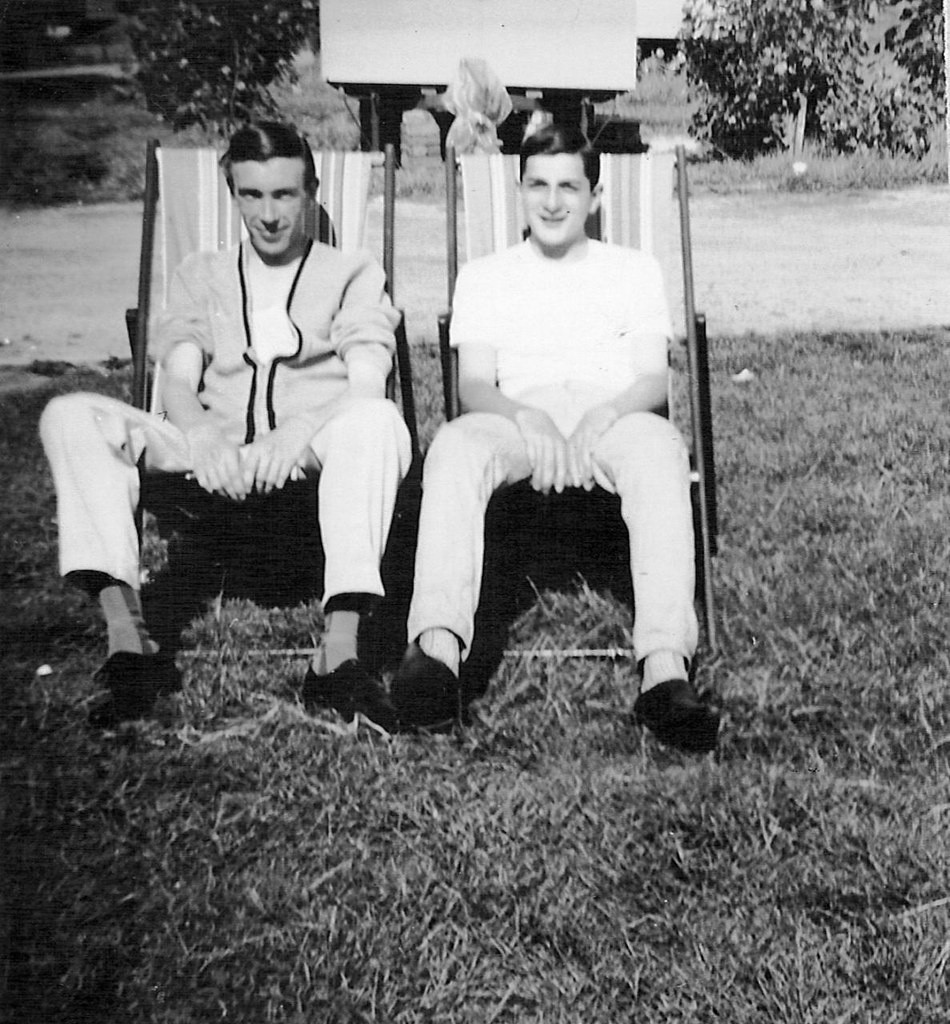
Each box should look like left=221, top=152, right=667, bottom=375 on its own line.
left=388, top=122, right=720, bottom=754
left=35, top=122, right=413, bottom=735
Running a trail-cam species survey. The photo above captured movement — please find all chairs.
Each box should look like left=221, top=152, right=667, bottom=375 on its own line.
left=128, top=137, right=414, bottom=656
left=439, top=145, right=722, bottom=663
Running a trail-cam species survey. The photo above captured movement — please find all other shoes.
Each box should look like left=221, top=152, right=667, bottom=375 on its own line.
left=87, top=644, right=184, bottom=728
left=300, top=658, right=394, bottom=729
left=389, top=639, right=462, bottom=724
left=633, top=679, right=720, bottom=749
